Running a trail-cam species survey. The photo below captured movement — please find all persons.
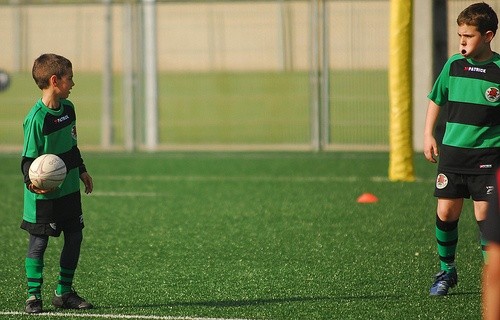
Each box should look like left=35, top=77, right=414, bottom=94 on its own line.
left=18, top=53, right=93, bottom=314
left=422, top=1, right=499, bottom=297
left=480, top=169, right=500, bottom=320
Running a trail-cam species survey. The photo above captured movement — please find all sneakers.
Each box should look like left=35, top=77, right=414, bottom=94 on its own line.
left=430, top=270, right=457, bottom=296
left=52, top=289, right=94, bottom=309
left=25, top=300, right=42, bottom=313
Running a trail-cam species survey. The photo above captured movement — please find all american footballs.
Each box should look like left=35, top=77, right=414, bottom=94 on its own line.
left=28, top=155, right=67, bottom=191
